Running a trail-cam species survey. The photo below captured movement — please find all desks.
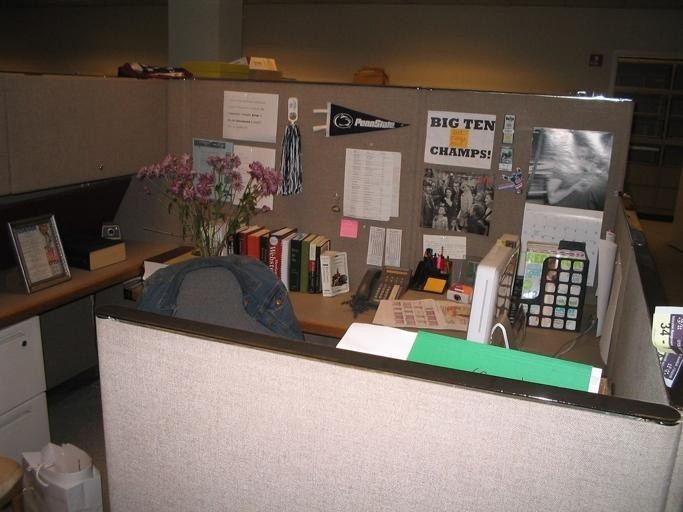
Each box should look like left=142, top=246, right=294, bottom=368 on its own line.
left=126, top=248, right=606, bottom=376
left=0, top=239, right=178, bottom=468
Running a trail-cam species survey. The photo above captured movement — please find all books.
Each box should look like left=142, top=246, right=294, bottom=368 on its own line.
left=520, top=239, right=588, bottom=332
left=200, top=216, right=350, bottom=298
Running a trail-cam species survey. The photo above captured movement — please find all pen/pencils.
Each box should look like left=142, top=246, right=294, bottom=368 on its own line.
left=424, top=246, right=452, bottom=274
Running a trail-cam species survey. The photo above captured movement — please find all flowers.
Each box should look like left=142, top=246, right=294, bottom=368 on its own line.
left=136, top=151, right=284, bottom=256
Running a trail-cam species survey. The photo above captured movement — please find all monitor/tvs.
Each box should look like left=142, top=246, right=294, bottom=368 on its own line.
left=466, top=232, right=522, bottom=348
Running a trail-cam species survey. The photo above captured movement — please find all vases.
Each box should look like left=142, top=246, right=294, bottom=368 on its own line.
left=195, top=240, right=222, bottom=256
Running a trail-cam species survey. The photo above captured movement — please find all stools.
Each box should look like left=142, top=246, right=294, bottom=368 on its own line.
left=0, top=455, right=25, bottom=512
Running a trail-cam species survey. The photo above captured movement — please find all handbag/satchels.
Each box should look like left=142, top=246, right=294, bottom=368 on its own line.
left=20, top=441, right=104, bottom=512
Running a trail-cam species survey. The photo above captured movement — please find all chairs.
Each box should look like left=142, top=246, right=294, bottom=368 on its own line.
left=138, top=254, right=305, bottom=343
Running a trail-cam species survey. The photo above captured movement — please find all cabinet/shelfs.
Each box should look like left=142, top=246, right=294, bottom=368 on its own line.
left=606, top=51, right=683, bottom=217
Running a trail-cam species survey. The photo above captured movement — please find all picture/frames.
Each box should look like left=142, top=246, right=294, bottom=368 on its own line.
left=6, top=212, right=72, bottom=294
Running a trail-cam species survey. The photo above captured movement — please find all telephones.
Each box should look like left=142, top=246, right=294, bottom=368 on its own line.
left=356, top=266, right=412, bottom=306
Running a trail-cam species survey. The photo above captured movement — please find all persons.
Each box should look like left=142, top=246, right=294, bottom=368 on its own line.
left=546, top=144, right=589, bottom=209
left=418, top=167, right=493, bottom=234
